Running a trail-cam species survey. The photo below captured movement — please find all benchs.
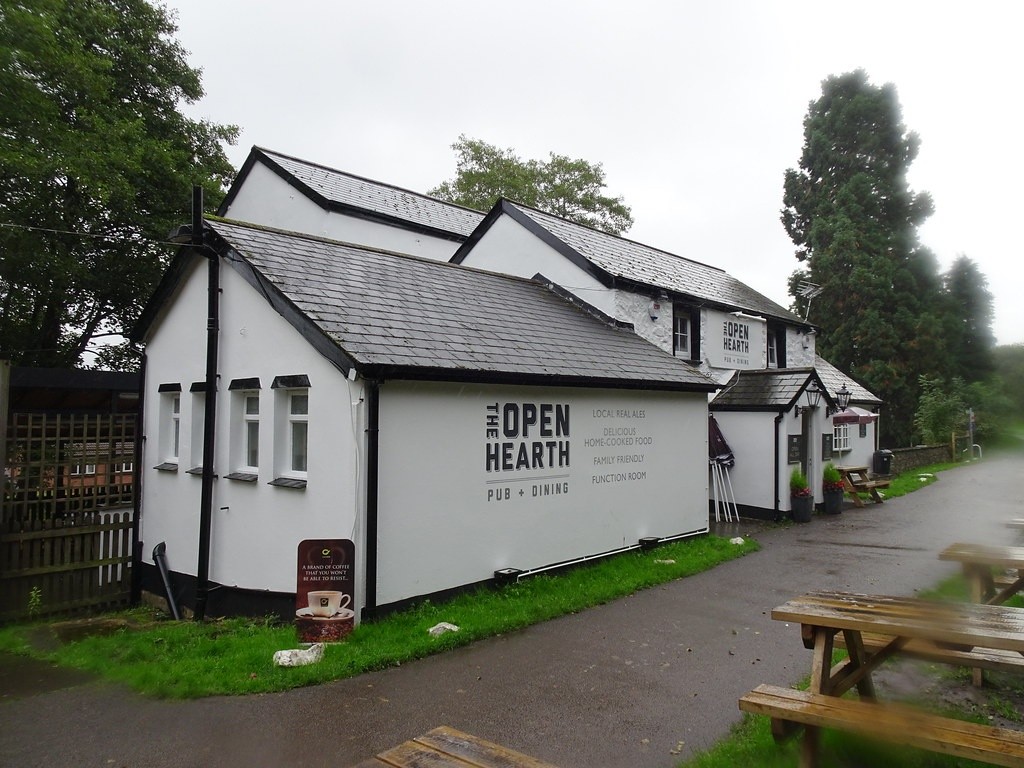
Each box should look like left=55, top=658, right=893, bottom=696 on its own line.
left=991, top=574, right=1020, bottom=589
left=833, top=630, right=1024, bottom=673
left=738, top=683, right=1024, bottom=768
left=853, top=479, right=892, bottom=492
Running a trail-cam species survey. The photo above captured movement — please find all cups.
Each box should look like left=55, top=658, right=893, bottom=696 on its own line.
left=307, top=590, right=351, bottom=617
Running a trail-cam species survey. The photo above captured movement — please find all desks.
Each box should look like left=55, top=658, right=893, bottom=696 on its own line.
left=770, top=588, right=1024, bottom=768
left=936, top=541, right=1024, bottom=687
left=837, top=465, right=883, bottom=508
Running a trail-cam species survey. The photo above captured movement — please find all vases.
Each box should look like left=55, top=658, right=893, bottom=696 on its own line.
left=822, top=490, right=844, bottom=515
left=790, top=496, right=814, bottom=524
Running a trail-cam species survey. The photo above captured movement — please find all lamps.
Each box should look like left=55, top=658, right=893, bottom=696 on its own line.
left=794, top=379, right=823, bottom=419
left=825, top=382, right=853, bottom=419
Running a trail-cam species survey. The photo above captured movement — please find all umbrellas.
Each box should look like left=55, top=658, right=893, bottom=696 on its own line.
left=709, top=413, right=740, bottom=522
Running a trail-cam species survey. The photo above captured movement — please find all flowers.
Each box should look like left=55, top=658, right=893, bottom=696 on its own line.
left=822, top=460, right=846, bottom=492
left=789, top=464, right=812, bottom=497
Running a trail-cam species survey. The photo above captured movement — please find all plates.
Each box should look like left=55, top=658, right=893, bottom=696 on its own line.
left=296, top=607, right=354, bottom=620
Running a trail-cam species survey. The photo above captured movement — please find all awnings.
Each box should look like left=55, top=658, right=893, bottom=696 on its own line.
left=833, top=406, right=881, bottom=426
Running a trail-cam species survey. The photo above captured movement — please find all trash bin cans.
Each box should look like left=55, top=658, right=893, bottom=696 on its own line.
left=873, top=449, right=893, bottom=474
